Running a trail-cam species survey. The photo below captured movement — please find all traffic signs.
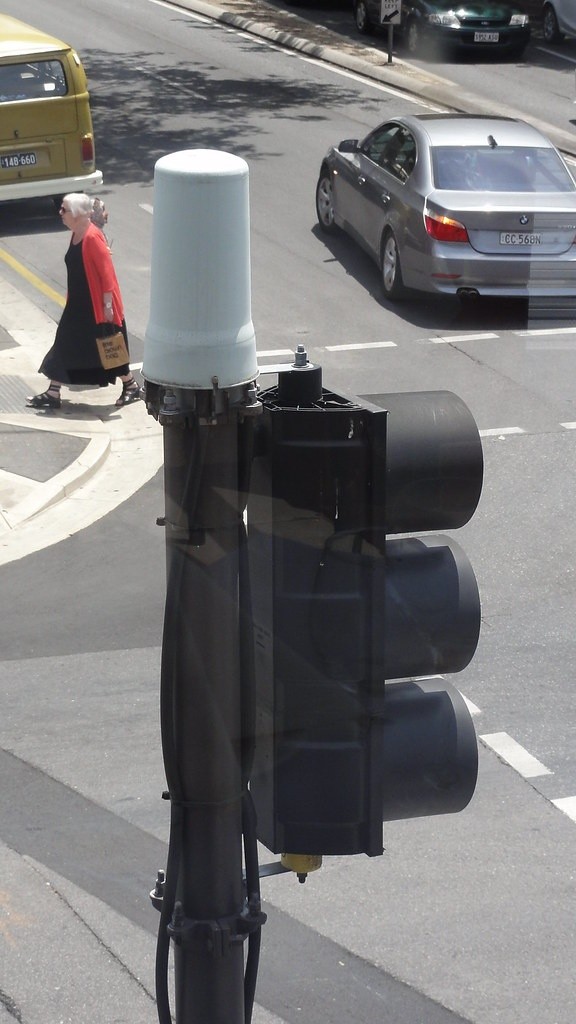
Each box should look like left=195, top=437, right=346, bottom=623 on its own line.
left=381, top=0, right=401, bottom=24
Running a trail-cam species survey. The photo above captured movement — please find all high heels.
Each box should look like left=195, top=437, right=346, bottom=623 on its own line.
left=115, top=377, right=142, bottom=406
left=25, top=385, right=62, bottom=409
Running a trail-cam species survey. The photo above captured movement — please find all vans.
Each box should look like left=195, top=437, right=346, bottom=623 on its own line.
left=0, top=12, right=104, bottom=215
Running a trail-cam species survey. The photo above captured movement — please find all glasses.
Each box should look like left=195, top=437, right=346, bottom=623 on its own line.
left=59, top=205, right=72, bottom=214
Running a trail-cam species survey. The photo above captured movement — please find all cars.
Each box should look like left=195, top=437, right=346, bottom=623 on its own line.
left=351, top=0, right=530, bottom=63
left=313, top=112, right=575, bottom=320
left=542, top=0, right=576, bottom=46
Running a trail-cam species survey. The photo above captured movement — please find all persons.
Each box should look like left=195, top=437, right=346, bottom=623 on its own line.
left=25, top=193, right=145, bottom=408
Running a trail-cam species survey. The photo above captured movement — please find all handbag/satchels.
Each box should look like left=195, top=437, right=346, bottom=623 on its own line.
left=95, top=320, right=131, bottom=370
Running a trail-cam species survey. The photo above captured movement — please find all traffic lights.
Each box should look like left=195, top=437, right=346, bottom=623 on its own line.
left=245, top=383, right=482, bottom=862
